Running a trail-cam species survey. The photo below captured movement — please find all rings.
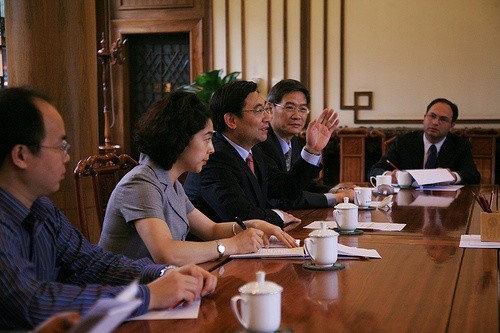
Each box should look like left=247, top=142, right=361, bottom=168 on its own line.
left=281, top=232, right=285, bottom=235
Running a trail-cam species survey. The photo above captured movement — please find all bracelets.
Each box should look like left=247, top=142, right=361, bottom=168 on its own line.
left=305, top=149, right=320, bottom=156
left=160, top=266, right=175, bottom=277
left=232, top=222, right=240, bottom=236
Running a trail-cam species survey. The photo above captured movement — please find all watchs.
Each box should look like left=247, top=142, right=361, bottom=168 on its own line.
left=215, top=240, right=225, bottom=258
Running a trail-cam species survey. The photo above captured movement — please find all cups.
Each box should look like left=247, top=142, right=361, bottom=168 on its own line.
left=304, top=221, right=339, bottom=267
left=369, top=175, right=392, bottom=192
left=395, top=172, right=412, bottom=188
left=230, top=271, right=283, bottom=333
left=333, top=197, right=359, bottom=233
left=480, top=210, right=500, bottom=243
left=354, top=187, right=372, bottom=208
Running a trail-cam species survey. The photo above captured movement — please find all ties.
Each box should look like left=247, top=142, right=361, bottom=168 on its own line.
left=425, top=145, right=437, bottom=169
left=286, top=149, right=292, bottom=172
left=247, top=155, right=254, bottom=177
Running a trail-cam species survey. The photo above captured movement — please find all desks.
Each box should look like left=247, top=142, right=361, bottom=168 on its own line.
left=109, top=184, right=500, bottom=333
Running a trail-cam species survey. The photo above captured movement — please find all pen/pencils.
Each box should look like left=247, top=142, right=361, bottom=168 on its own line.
left=472, top=190, right=495, bottom=214
left=386, top=160, right=399, bottom=171
left=235, top=216, right=248, bottom=230
left=167, top=296, right=186, bottom=314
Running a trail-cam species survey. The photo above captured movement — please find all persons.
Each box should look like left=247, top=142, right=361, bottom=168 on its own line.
left=96, top=92, right=299, bottom=267
left=368, top=97, right=481, bottom=186
left=0, top=87, right=219, bottom=333
left=185, top=78, right=360, bottom=245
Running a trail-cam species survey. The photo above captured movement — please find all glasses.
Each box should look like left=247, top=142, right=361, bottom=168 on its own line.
left=233, top=102, right=273, bottom=118
left=273, top=102, right=310, bottom=113
left=26, top=144, right=70, bottom=155
